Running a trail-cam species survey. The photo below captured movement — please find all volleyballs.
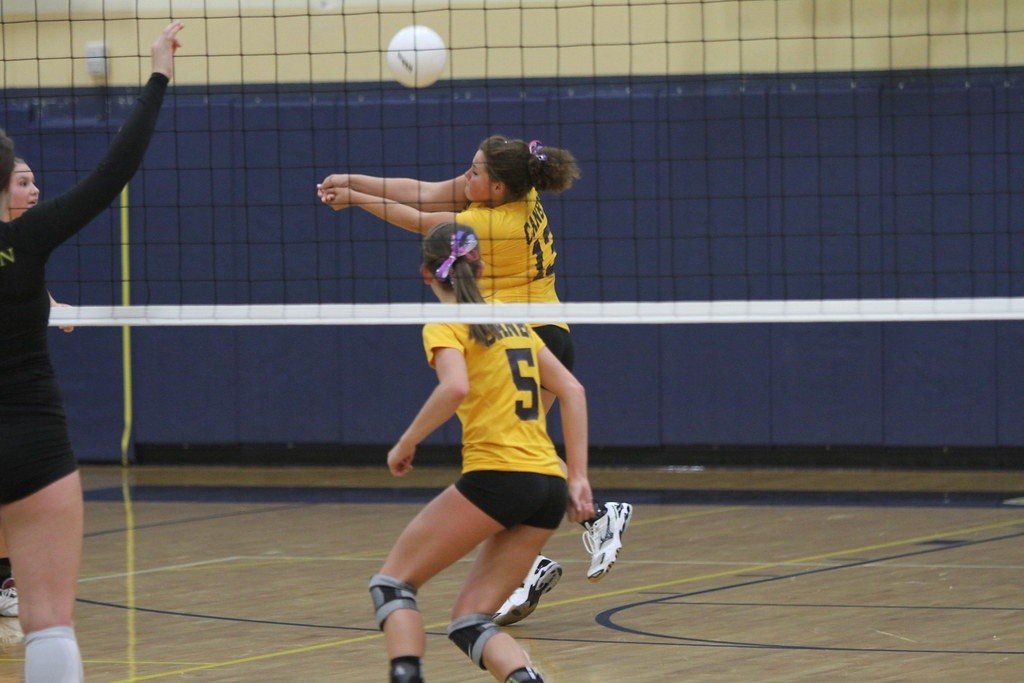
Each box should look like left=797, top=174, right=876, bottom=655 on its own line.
left=387, top=25, right=446, bottom=89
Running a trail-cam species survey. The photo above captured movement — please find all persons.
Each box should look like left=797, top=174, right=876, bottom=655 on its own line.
left=316, top=135, right=632, bottom=626
left=0, top=22, right=182, bottom=683
left=369, top=223, right=594, bottom=683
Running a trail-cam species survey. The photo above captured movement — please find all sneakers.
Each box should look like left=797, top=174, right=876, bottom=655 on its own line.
left=491, top=555, right=563, bottom=626
left=0, top=578, right=19, bottom=616
left=582, top=501, right=633, bottom=582
